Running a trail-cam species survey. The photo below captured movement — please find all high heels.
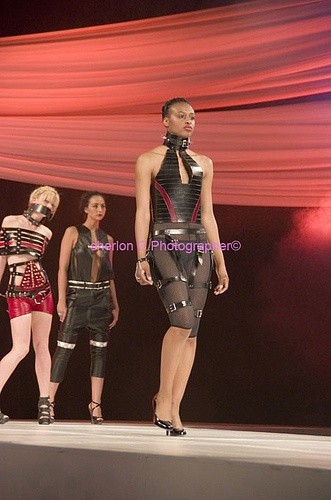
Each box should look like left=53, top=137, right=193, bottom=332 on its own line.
left=88, top=401, right=104, bottom=424
left=153, top=392, right=186, bottom=436
left=38, top=395, right=51, bottom=425
left=0, top=412, right=9, bottom=424
left=48, top=401, right=55, bottom=424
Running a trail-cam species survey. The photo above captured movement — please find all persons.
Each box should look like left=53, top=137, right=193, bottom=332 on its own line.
left=134, top=97, right=229, bottom=437
left=0, top=185, right=60, bottom=425
left=48, top=189, right=120, bottom=423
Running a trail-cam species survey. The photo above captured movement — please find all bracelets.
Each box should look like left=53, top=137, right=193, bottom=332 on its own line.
left=137, top=257, right=149, bottom=263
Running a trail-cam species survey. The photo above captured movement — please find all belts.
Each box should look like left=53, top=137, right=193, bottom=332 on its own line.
left=7, top=287, right=51, bottom=303
left=68, top=279, right=110, bottom=290
left=151, top=227, right=206, bottom=237
